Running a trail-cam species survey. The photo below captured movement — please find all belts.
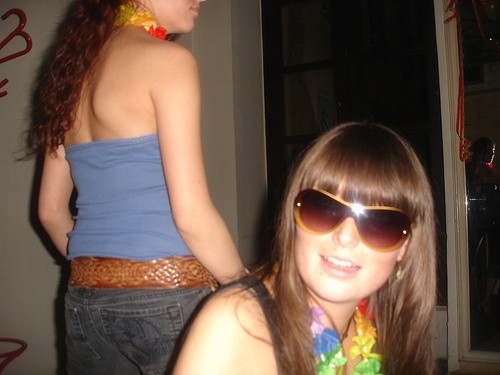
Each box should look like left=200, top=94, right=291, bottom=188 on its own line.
left=67, top=255, right=218, bottom=290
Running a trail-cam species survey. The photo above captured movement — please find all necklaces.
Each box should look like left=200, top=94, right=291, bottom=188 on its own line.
left=306, top=292, right=381, bottom=375
left=113, top=5, right=168, bottom=39
left=340, top=309, right=356, bottom=344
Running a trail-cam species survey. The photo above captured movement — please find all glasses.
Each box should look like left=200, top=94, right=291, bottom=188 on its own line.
left=293, top=190, right=412, bottom=252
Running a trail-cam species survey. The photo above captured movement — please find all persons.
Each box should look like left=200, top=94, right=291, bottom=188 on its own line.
left=19, top=0, right=253, bottom=375
left=467, top=137, right=499, bottom=213
left=168, top=121, right=440, bottom=375
